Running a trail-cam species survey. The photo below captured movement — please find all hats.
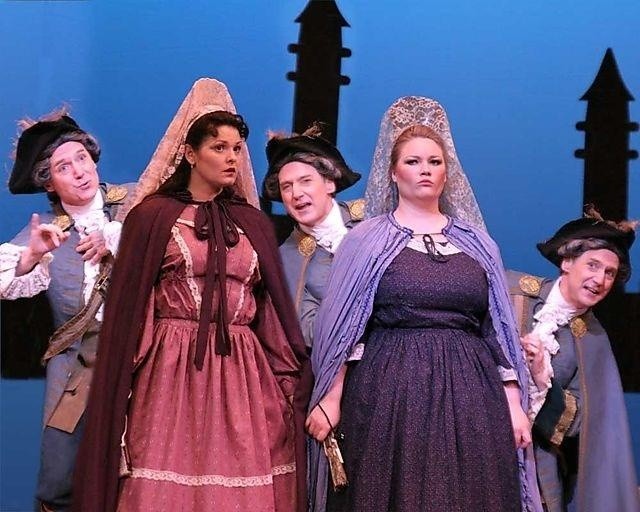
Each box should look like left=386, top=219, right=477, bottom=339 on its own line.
left=9, top=116, right=100, bottom=196
left=534, top=218, right=636, bottom=268
left=259, top=133, right=362, bottom=202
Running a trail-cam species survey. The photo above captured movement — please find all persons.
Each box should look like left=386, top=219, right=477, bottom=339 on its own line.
left=71, top=77, right=310, bottom=511
left=259, top=119, right=365, bottom=340
left=307, top=94, right=543, bottom=512
left=504, top=207, right=640, bottom=511
left=1, top=104, right=136, bottom=512
